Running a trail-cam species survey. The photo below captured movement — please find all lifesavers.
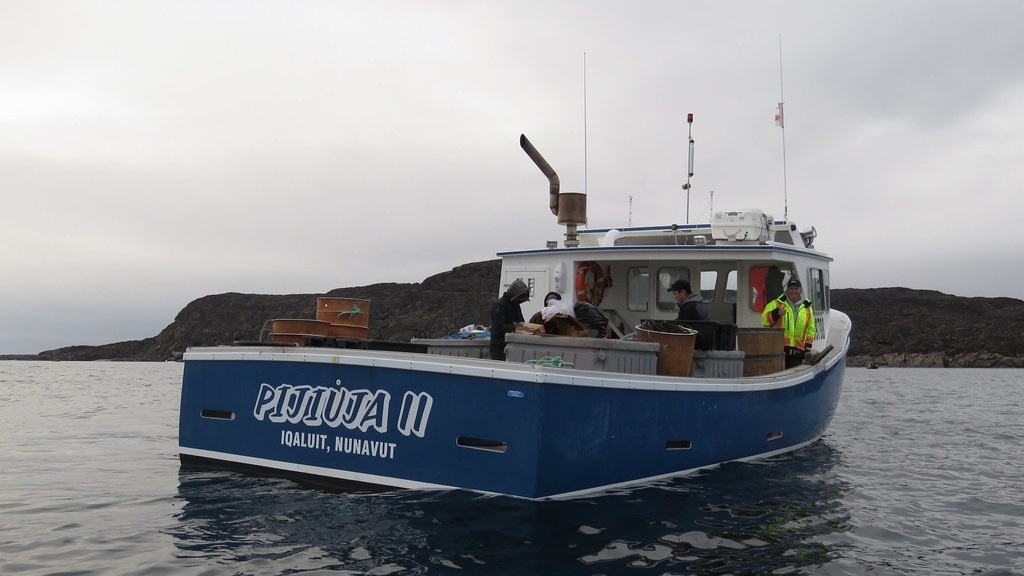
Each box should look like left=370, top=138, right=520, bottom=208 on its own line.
left=575, top=261, right=605, bottom=307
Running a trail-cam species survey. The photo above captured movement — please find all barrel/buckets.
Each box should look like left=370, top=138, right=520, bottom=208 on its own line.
left=634, top=319, right=698, bottom=376
left=316, top=296, right=370, bottom=338
left=257, top=318, right=330, bottom=349
left=738, top=328, right=785, bottom=377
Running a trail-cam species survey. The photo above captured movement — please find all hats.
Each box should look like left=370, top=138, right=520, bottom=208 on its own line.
left=667, top=280, right=691, bottom=292
left=787, top=279, right=801, bottom=288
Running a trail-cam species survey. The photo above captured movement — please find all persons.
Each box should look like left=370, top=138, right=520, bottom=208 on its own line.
left=489, top=279, right=530, bottom=361
left=529, top=289, right=562, bottom=325
left=762, top=279, right=815, bottom=369
left=666, top=279, right=711, bottom=320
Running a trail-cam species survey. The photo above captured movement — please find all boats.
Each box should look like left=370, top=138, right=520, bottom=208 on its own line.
left=175, top=48, right=853, bottom=509
